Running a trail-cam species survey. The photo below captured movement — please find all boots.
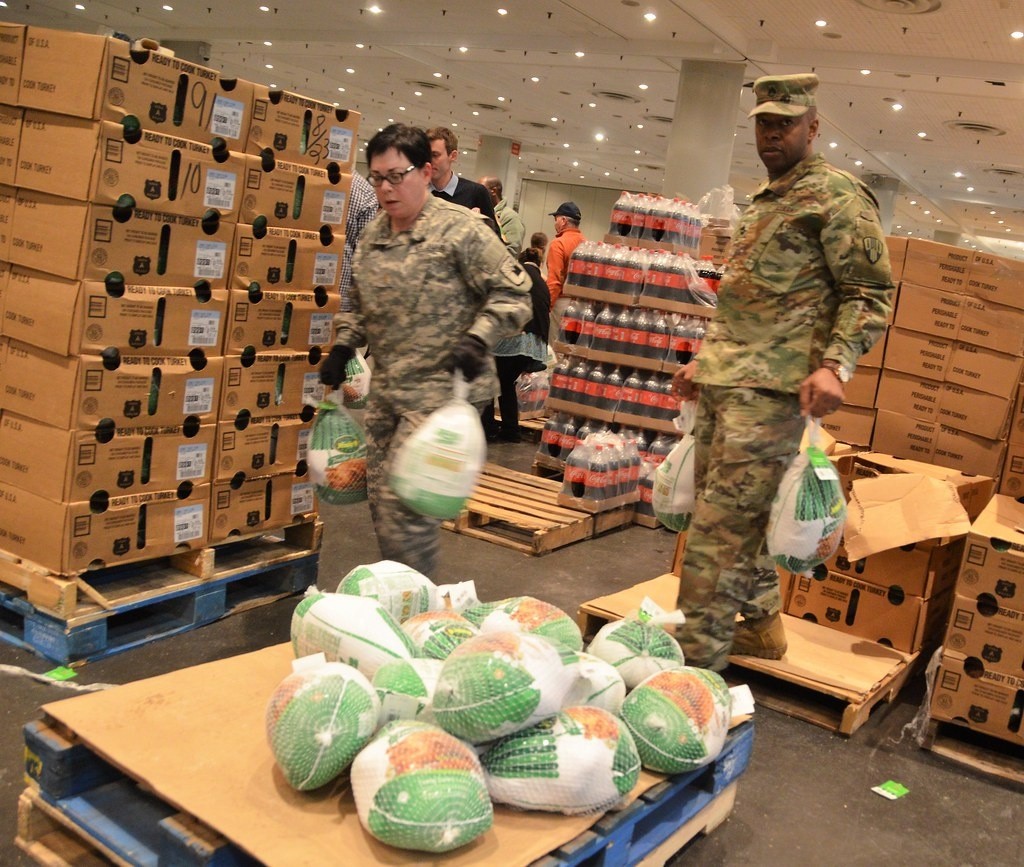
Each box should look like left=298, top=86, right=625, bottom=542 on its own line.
left=731, top=611, right=787, bottom=659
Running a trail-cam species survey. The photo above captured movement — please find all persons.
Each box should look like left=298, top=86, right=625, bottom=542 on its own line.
left=677, top=74, right=894, bottom=675
left=482, top=176, right=527, bottom=258
left=532, top=233, right=548, bottom=279
left=546, top=201, right=586, bottom=357
left=427, top=127, right=501, bottom=239
left=319, top=123, right=533, bottom=578
left=481, top=251, right=556, bottom=445
left=341, top=168, right=379, bottom=312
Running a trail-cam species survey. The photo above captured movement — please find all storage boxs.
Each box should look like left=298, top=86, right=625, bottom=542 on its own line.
left=0, top=22, right=363, bottom=577
left=671, top=234, right=1024, bottom=749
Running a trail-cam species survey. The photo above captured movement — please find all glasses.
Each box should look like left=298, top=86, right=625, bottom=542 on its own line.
left=367, top=164, right=416, bottom=187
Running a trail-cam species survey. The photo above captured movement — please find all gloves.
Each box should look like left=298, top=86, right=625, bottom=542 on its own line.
left=318, top=345, right=357, bottom=389
left=442, top=334, right=486, bottom=382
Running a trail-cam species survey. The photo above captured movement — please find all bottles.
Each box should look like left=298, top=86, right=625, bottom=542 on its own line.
left=608, top=190, right=703, bottom=250
left=537, top=410, right=685, bottom=517
left=548, top=354, right=688, bottom=421
left=566, top=239, right=729, bottom=306
left=558, top=297, right=712, bottom=366
left=514, top=369, right=552, bottom=413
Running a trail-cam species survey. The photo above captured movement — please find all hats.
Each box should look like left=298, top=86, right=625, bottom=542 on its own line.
left=747, top=73, right=819, bottom=118
left=548, top=202, right=581, bottom=221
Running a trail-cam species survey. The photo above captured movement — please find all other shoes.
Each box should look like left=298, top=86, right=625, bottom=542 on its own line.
left=501, top=429, right=521, bottom=443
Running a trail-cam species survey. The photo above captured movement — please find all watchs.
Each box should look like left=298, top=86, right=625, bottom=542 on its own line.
left=822, top=361, right=849, bottom=382
